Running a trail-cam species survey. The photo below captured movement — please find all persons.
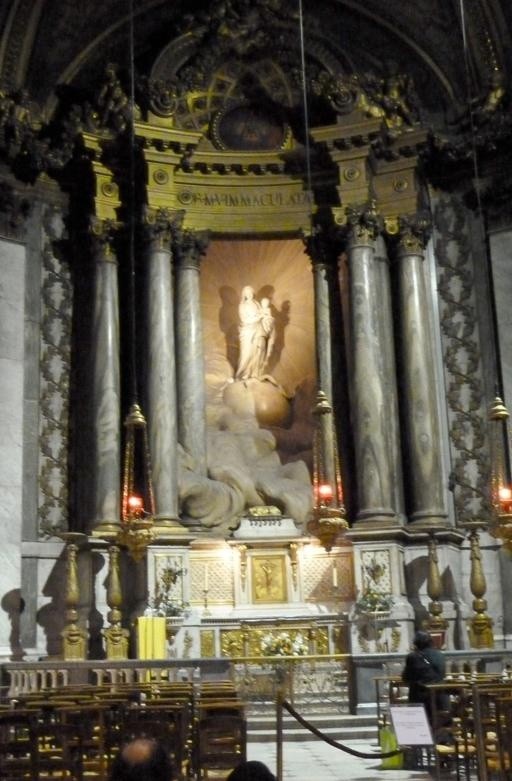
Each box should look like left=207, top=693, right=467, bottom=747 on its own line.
left=401, top=631, right=458, bottom=767
left=107, top=735, right=173, bottom=780
left=237, top=286, right=278, bottom=380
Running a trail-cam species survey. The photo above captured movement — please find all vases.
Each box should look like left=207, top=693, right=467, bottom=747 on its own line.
left=370, top=612, right=391, bottom=632
left=163, top=618, right=184, bottom=640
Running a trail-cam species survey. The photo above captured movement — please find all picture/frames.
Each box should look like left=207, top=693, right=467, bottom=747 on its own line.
left=250, top=555, right=290, bottom=605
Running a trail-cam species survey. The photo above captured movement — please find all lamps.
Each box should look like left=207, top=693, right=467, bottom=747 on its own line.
left=457, top=9, right=511, bottom=554
left=118, top=0, right=157, bottom=561
left=295, top=0, right=350, bottom=552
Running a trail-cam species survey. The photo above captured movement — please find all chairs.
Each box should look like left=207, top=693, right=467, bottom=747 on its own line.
left=1, top=681, right=248, bottom=781
left=391, top=672, right=509, bottom=781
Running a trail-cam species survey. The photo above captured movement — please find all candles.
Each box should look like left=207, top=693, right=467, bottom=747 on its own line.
left=202, top=564, right=212, bottom=617
left=331, top=560, right=340, bottom=611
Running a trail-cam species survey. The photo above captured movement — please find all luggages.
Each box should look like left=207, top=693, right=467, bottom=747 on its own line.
left=378, top=712, right=423, bottom=770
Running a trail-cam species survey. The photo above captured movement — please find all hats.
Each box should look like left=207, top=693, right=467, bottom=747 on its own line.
left=415, top=630, right=431, bottom=642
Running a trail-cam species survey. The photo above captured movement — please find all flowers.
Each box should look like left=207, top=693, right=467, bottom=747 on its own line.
left=254, top=630, right=309, bottom=668
left=350, top=571, right=401, bottom=612
left=137, top=559, right=196, bottom=617
